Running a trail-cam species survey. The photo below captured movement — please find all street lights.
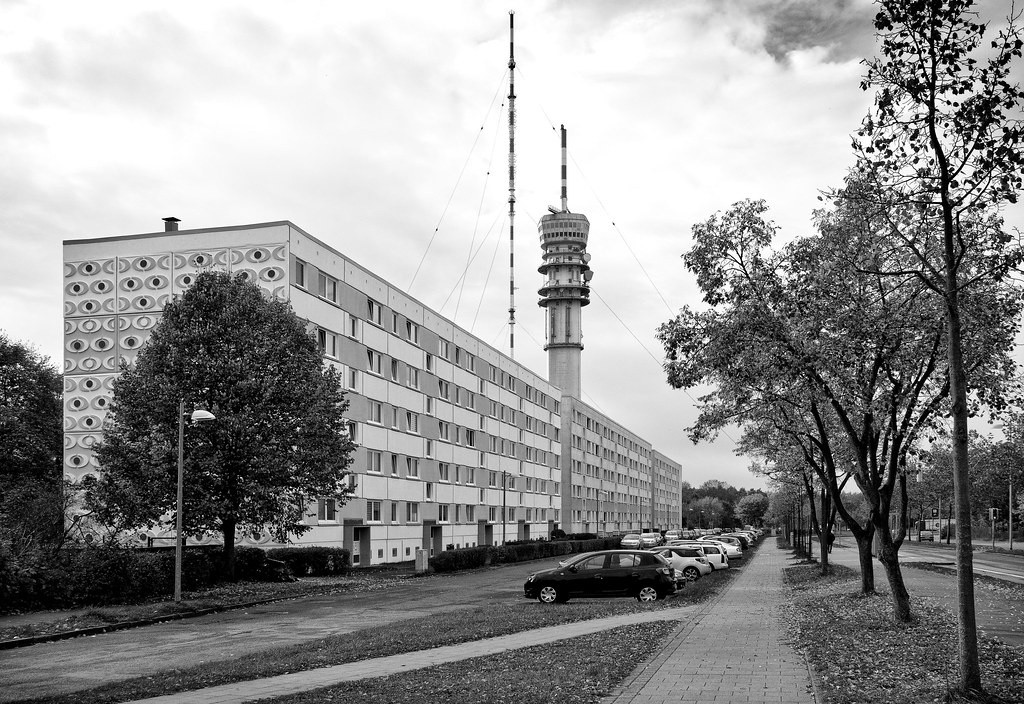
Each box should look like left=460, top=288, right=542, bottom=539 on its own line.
left=699, top=510, right=705, bottom=529
left=502, top=470, right=521, bottom=561
left=174, top=403, right=216, bottom=600
left=991, top=423, right=1024, bottom=550
left=596, top=489, right=608, bottom=540
left=687, top=507, right=693, bottom=530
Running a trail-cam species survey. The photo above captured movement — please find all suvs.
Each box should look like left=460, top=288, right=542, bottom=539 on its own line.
left=637, top=546, right=710, bottom=581
left=663, top=529, right=766, bottom=575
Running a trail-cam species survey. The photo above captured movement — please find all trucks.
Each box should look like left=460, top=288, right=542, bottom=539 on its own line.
left=915, top=519, right=957, bottom=531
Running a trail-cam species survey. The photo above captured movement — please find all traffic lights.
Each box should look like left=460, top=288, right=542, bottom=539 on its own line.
left=993, top=509, right=997, bottom=520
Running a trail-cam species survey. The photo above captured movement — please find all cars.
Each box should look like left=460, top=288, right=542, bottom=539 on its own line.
left=641, top=532, right=663, bottom=547
left=620, top=534, right=642, bottom=549
left=664, top=528, right=732, bottom=540
left=919, top=531, right=933, bottom=542
left=523, top=550, right=686, bottom=603
